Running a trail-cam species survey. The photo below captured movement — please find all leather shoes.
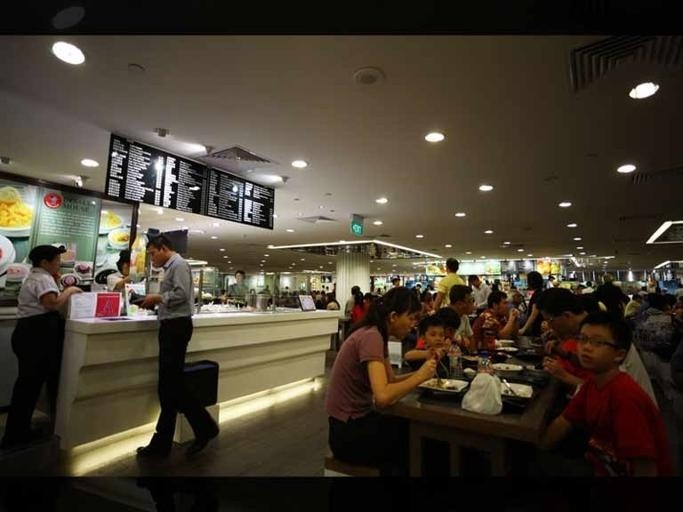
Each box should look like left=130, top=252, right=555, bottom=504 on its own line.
left=185, top=428, right=220, bottom=454
left=137, top=447, right=146, bottom=457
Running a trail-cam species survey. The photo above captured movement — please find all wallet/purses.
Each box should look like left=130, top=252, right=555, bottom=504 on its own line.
left=130, top=293, right=156, bottom=312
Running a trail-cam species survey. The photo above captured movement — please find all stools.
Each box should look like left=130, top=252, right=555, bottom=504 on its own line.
left=319, top=456, right=383, bottom=480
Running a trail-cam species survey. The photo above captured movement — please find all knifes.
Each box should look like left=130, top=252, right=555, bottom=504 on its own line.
left=431, top=365, right=445, bottom=387
left=501, top=377, right=522, bottom=398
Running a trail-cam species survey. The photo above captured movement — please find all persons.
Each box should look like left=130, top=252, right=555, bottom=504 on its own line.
left=536, top=288, right=662, bottom=415
left=447, top=285, right=478, bottom=354
left=472, top=291, right=521, bottom=346
left=404, top=315, right=450, bottom=377
left=432, top=307, right=464, bottom=355
left=263, top=283, right=340, bottom=310
left=325, top=287, right=437, bottom=478
left=107, top=249, right=132, bottom=315
left=344, top=276, right=437, bottom=324
left=218, top=270, right=249, bottom=300
left=1, top=245, right=84, bottom=449
left=433, top=259, right=465, bottom=309
left=468, top=271, right=683, bottom=313
left=534, top=312, right=675, bottom=478
left=129, top=236, right=219, bottom=456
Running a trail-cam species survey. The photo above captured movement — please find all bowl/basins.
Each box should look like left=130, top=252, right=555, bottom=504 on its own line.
left=526, top=362, right=554, bottom=387
left=489, top=361, right=522, bottom=380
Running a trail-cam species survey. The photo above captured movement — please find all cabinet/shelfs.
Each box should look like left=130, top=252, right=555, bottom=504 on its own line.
left=52, top=308, right=344, bottom=476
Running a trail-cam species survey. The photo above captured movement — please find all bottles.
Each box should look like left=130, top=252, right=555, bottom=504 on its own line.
left=481, top=316, right=495, bottom=357
left=476, top=352, right=493, bottom=376
left=449, top=339, right=462, bottom=380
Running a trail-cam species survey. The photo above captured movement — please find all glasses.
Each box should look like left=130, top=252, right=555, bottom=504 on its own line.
left=462, top=297, right=475, bottom=306
left=545, top=316, right=554, bottom=325
left=574, top=334, right=618, bottom=349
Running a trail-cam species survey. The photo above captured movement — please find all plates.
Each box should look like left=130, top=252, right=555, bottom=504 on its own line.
left=492, top=345, right=519, bottom=351
left=494, top=338, right=517, bottom=345
left=417, top=377, right=468, bottom=393
left=514, top=352, right=552, bottom=363
left=438, top=367, right=475, bottom=381
left=497, top=381, right=533, bottom=400
left=471, top=349, right=508, bottom=365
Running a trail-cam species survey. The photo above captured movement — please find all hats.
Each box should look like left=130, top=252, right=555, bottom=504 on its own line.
left=28, top=245, right=67, bottom=260
